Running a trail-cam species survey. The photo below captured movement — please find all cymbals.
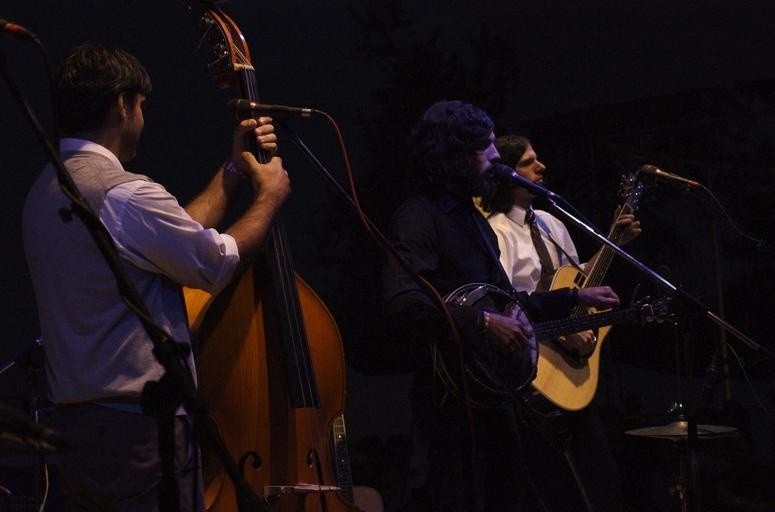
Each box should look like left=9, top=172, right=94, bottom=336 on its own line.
left=625, top=422, right=738, bottom=441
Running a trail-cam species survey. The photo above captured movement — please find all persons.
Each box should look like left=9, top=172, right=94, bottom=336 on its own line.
left=380, top=100, right=623, bottom=511
left=19, top=41, right=293, bottom=512
left=478, top=135, right=646, bottom=509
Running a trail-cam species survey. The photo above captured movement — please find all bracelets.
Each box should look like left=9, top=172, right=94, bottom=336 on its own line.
left=481, top=311, right=491, bottom=337
left=220, top=159, right=246, bottom=183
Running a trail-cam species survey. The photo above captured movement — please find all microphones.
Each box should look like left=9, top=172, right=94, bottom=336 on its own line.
left=489, top=159, right=560, bottom=200
left=221, top=96, right=315, bottom=125
left=640, top=160, right=704, bottom=193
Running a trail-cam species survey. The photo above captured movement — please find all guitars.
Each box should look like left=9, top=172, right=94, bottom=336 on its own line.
left=522, top=171, right=647, bottom=418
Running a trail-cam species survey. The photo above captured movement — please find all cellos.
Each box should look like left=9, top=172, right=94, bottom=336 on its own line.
left=179, top=6, right=381, bottom=512
